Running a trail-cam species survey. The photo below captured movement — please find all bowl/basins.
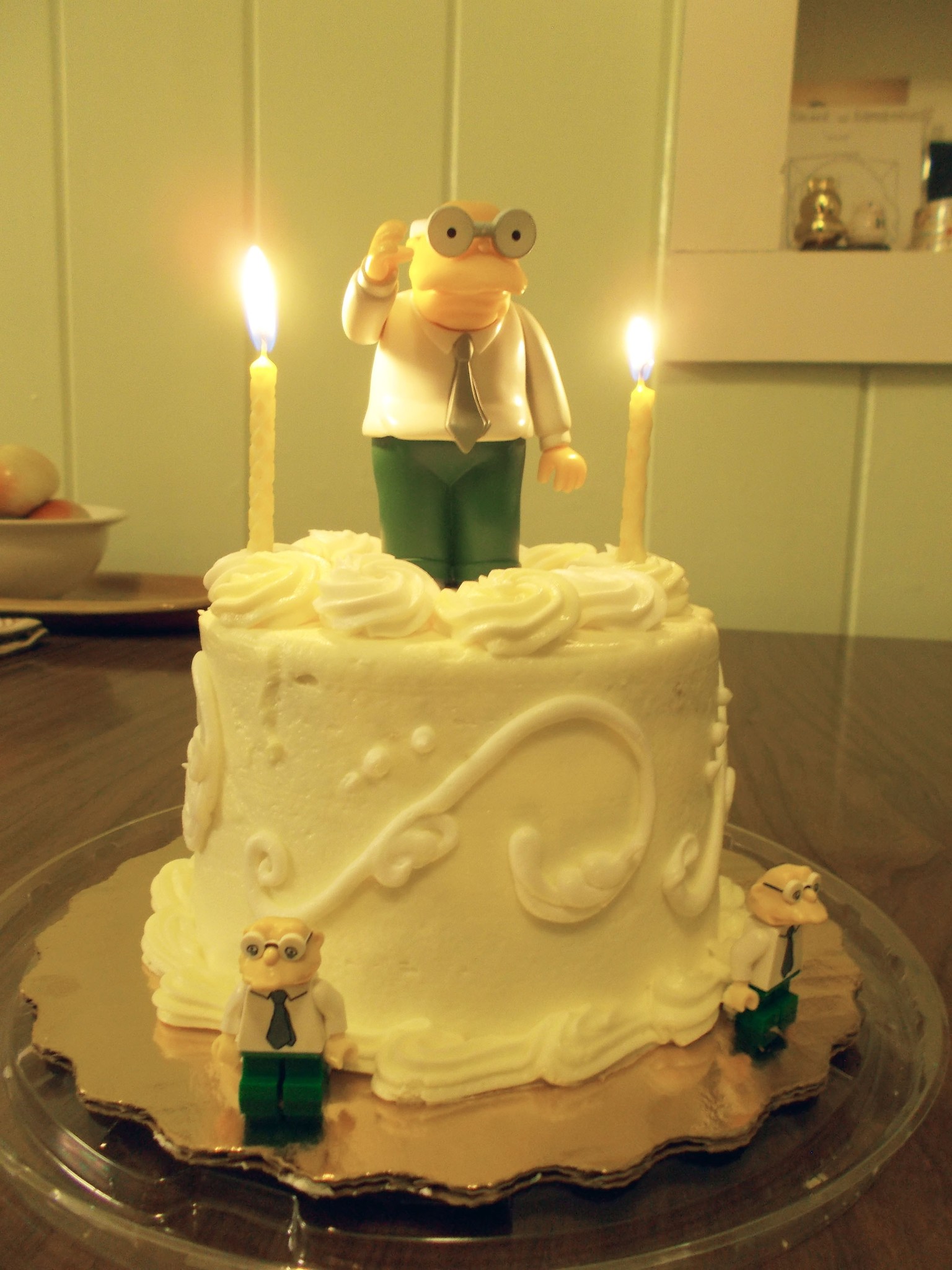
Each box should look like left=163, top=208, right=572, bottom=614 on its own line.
left=0, top=504, right=133, bottom=599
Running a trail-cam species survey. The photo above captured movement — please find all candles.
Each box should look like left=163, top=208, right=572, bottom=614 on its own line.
left=238, top=241, right=281, bottom=553
left=616, top=307, right=661, bottom=567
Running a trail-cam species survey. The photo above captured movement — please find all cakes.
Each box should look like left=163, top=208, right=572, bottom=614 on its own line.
left=135, top=518, right=766, bottom=1118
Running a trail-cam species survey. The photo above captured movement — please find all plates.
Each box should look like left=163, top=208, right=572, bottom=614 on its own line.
left=0, top=571, right=216, bottom=616
left=1, top=793, right=952, bottom=1268
